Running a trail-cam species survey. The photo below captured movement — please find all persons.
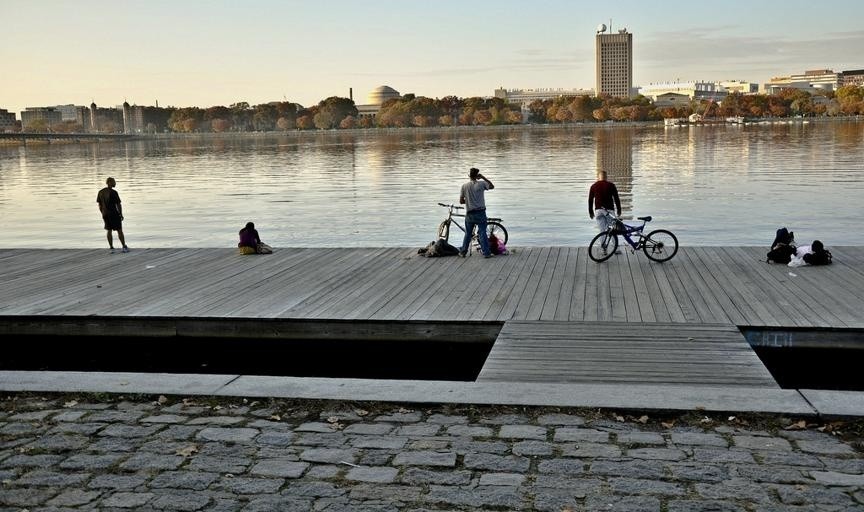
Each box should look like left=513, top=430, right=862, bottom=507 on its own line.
left=237, top=221, right=260, bottom=255
left=457, top=168, right=495, bottom=258
left=95, top=176, right=130, bottom=254
left=587, top=171, right=622, bottom=254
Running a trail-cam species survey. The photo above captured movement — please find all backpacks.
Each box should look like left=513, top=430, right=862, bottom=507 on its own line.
left=766, top=227, right=833, bottom=268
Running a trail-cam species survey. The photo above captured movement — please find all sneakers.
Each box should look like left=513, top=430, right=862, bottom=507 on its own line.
left=458, top=251, right=494, bottom=258
left=110, top=245, right=129, bottom=254
left=601, top=249, right=621, bottom=256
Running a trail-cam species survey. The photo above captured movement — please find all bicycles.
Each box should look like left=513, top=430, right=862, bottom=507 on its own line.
left=588, top=206, right=678, bottom=264
left=436, top=203, right=508, bottom=256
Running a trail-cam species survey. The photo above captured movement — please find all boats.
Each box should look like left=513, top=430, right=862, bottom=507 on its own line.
left=674, top=120, right=794, bottom=128
left=802, top=121, right=810, bottom=124
